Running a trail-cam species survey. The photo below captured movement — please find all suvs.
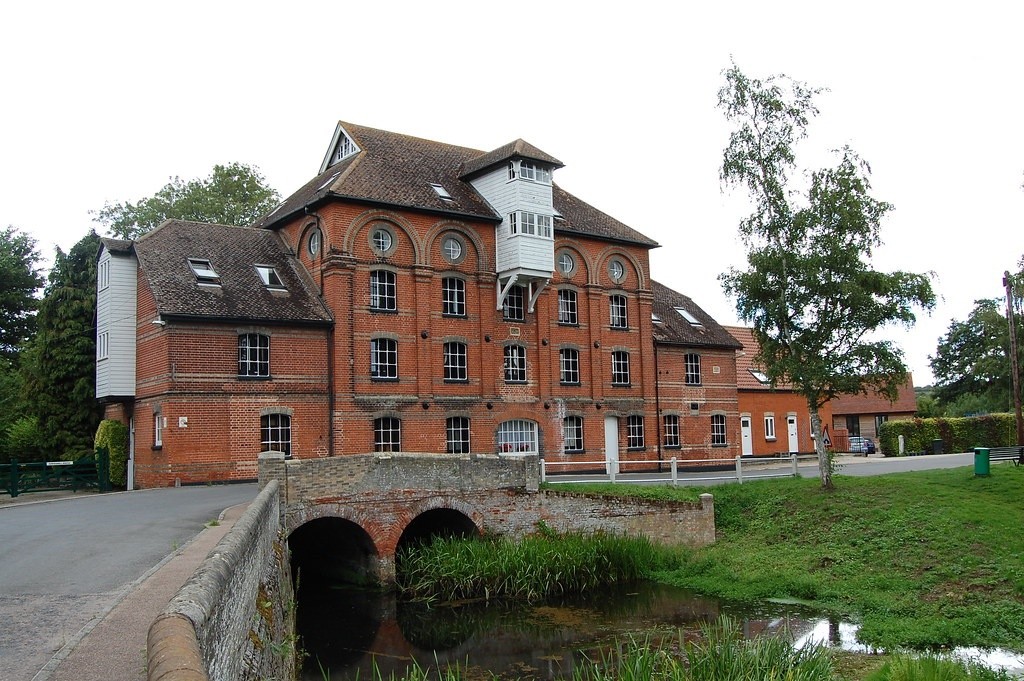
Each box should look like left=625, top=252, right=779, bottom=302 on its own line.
left=849, top=436, right=868, bottom=453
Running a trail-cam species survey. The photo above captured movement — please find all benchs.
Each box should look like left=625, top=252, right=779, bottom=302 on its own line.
left=989, top=445, right=1024, bottom=466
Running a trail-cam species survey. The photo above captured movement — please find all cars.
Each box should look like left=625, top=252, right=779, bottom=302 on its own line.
left=863, top=438, right=875, bottom=454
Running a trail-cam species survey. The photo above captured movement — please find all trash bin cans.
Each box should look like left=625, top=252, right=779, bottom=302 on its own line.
left=932, top=439, right=943, bottom=455
left=975, top=448, right=992, bottom=477
